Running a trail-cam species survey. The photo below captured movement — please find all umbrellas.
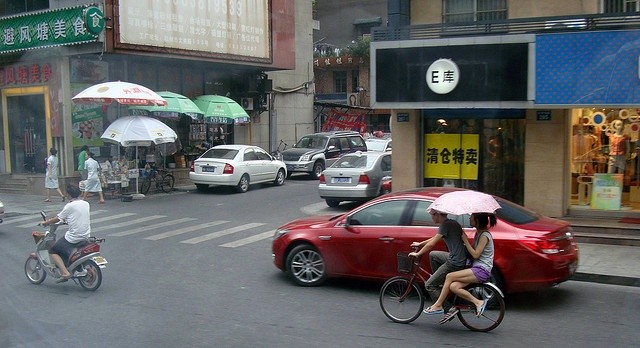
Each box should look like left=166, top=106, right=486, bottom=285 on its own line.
left=100, top=115, right=178, bottom=194
left=426, top=190, right=502, bottom=246
left=128, top=91, right=204, bottom=170
left=70, top=80, right=168, bottom=161
left=194, top=94, right=251, bottom=154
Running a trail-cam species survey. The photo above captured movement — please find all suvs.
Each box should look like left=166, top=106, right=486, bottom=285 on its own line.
left=278, top=131, right=367, bottom=179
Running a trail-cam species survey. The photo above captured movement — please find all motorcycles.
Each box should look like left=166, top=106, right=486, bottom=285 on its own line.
left=25, top=210, right=107, bottom=291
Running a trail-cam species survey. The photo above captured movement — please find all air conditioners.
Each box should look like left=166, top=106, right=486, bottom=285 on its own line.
left=240, top=97, right=253, bottom=111
left=545, top=18, right=587, bottom=28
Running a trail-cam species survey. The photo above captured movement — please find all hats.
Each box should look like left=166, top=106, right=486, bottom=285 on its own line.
left=429, top=208, right=444, bottom=214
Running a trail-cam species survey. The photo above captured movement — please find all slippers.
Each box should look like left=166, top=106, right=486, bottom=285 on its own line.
left=423, top=306, right=444, bottom=315
left=476, top=299, right=488, bottom=318
left=56, top=274, right=72, bottom=284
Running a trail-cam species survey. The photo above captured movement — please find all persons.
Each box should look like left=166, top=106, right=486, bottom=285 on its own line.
left=82, top=152, right=106, bottom=204
left=78, top=145, right=90, bottom=197
left=44, top=147, right=65, bottom=202
left=504, top=138, right=519, bottom=197
left=607, top=120, right=630, bottom=205
left=487, top=134, right=500, bottom=161
left=38, top=184, right=91, bottom=283
left=408, top=209, right=468, bottom=325
left=422, top=212, right=497, bottom=318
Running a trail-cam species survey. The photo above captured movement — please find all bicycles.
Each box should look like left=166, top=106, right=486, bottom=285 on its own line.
left=379, top=246, right=505, bottom=332
left=141, top=165, right=174, bottom=193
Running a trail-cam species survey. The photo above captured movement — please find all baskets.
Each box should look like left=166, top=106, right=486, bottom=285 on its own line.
left=142, top=169, right=151, bottom=177
left=396, top=251, right=415, bottom=274
left=32, top=232, right=56, bottom=248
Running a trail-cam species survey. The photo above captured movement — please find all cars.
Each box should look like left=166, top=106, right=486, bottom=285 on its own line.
left=190, top=145, right=287, bottom=193
left=319, top=153, right=392, bottom=207
left=364, top=139, right=392, bottom=152
left=273, top=189, right=578, bottom=309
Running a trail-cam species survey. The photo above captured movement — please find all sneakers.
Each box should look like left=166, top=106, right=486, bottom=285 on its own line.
left=62, top=195, right=65, bottom=202
left=44, top=199, right=51, bottom=202
left=439, top=306, right=459, bottom=325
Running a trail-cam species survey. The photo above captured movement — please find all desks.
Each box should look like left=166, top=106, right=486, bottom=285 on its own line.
left=103, top=180, right=122, bottom=199
